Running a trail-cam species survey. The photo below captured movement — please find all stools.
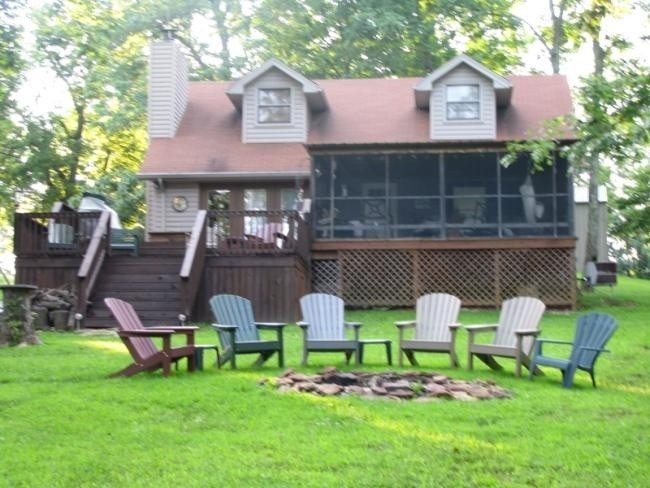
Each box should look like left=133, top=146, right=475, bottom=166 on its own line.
left=175, top=345, right=221, bottom=370
left=358, top=339, right=392, bottom=366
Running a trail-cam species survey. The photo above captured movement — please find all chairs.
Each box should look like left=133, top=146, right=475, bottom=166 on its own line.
left=530, top=312, right=622, bottom=390
left=393, top=292, right=462, bottom=370
left=207, top=293, right=288, bottom=370
left=465, top=296, right=547, bottom=377
left=296, top=292, right=363, bottom=365
left=103, top=296, right=199, bottom=377
